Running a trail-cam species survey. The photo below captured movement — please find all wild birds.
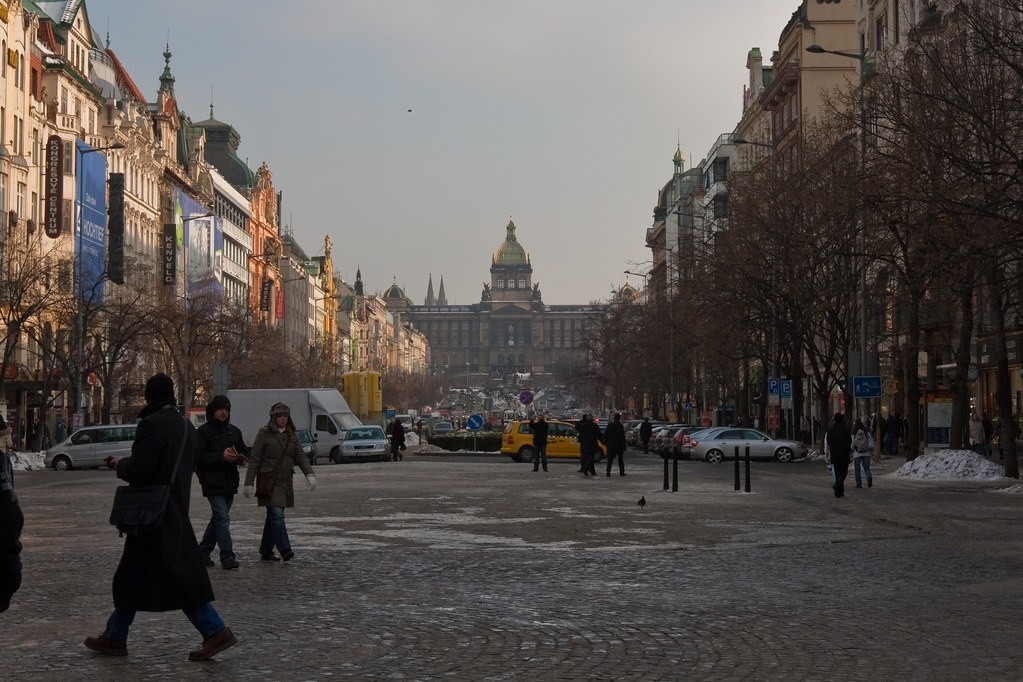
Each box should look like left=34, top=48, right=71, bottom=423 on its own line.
left=406, top=110, right=412, bottom=112
left=638, top=496, right=647, bottom=509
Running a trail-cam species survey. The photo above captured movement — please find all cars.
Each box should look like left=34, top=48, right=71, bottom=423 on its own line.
left=294, top=429, right=318, bottom=465
left=535, top=388, right=577, bottom=420
left=623, top=419, right=809, bottom=463
left=421, top=390, right=474, bottom=434
left=339, top=425, right=393, bottom=463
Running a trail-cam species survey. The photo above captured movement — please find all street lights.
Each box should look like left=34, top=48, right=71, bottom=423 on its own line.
left=733, top=138, right=778, bottom=437
left=313, top=295, right=424, bottom=374
left=673, top=210, right=706, bottom=252
left=243, top=251, right=274, bottom=358
left=623, top=270, right=646, bottom=309
left=180, top=211, right=216, bottom=418
left=77, top=142, right=124, bottom=412
left=645, top=244, right=676, bottom=410
left=805, top=42, right=869, bottom=430
left=277, top=274, right=306, bottom=359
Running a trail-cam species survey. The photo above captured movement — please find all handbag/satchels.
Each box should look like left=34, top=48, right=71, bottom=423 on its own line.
left=109, top=485, right=170, bottom=538
left=400, top=442, right=406, bottom=450
left=254, top=474, right=274, bottom=499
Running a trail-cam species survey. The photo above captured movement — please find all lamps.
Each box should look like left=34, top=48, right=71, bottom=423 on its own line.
left=2, top=140, right=13, bottom=146
left=10, top=150, right=32, bottom=157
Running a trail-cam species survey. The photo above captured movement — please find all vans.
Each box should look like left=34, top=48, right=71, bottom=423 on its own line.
left=499, top=420, right=607, bottom=462
left=44, top=424, right=138, bottom=471
left=394, top=415, right=412, bottom=432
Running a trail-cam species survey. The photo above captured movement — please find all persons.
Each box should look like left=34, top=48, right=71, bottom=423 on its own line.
left=604, top=412, right=628, bottom=476
left=968, top=412, right=1004, bottom=460
left=529, top=414, right=549, bottom=471
left=190, top=395, right=249, bottom=568
left=639, top=417, right=652, bottom=454
left=576, top=412, right=604, bottom=476
left=386, top=418, right=406, bottom=461
left=811, top=408, right=913, bottom=497
left=245, top=403, right=315, bottom=563
left=451, top=416, right=504, bottom=429
left=84, top=373, right=238, bottom=660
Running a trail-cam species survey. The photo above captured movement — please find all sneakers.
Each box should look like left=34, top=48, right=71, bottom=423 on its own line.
left=84, top=632, right=129, bottom=656
left=188, top=628, right=238, bottom=661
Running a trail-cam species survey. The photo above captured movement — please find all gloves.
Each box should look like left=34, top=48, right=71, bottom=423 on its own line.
left=243, top=485, right=253, bottom=498
left=306, top=474, right=317, bottom=491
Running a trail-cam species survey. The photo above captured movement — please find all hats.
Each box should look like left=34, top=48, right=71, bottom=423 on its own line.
left=210, top=395, right=230, bottom=416
left=145, top=373, right=174, bottom=403
left=270, top=402, right=290, bottom=416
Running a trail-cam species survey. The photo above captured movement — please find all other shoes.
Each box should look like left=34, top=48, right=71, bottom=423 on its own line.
left=868, top=478, right=872, bottom=487
left=855, top=482, right=862, bottom=489
left=543, top=469, right=549, bottom=472
left=262, top=552, right=281, bottom=561
left=200, top=554, right=215, bottom=566
left=833, top=484, right=844, bottom=498
left=281, top=549, right=294, bottom=561
left=577, top=467, right=627, bottom=477
left=222, top=557, right=240, bottom=569
left=532, top=469, right=538, bottom=472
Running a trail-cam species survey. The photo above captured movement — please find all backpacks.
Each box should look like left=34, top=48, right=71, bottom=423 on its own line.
left=854, top=429, right=868, bottom=453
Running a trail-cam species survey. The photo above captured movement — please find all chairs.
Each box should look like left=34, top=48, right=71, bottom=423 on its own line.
left=372, top=430, right=379, bottom=438
left=352, top=433, right=359, bottom=438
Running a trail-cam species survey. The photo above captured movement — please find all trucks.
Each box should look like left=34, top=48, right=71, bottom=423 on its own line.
left=539, top=400, right=547, bottom=408
left=228, top=388, right=363, bottom=464
